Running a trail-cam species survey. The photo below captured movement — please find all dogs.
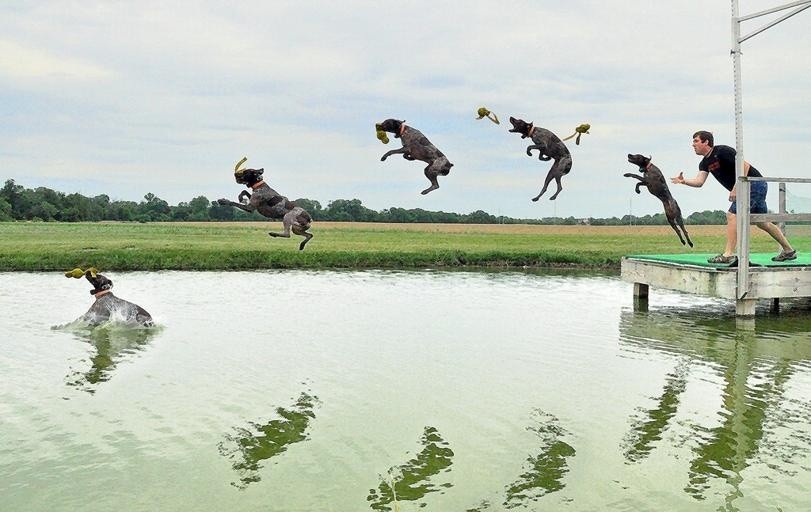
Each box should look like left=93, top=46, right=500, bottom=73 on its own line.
left=377, top=118, right=454, bottom=196
left=623, top=153, right=694, bottom=248
left=508, top=116, right=573, bottom=202
left=217, top=167, right=314, bottom=251
left=50, top=270, right=154, bottom=330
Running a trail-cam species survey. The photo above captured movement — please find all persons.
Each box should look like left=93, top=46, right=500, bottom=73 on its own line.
left=668, top=131, right=798, bottom=264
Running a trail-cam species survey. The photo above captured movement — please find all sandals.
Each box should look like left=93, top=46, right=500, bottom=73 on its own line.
left=771, top=250, right=797, bottom=262
left=707, top=253, right=737, bottom=263
